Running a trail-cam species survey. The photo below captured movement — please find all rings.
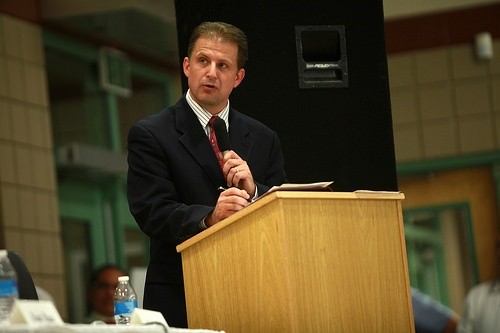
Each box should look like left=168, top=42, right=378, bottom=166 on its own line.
left=235, top=167, right=239, bottom=172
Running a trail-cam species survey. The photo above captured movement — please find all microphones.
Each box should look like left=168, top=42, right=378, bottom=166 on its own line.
left=213, top=118, right=235, bottom=187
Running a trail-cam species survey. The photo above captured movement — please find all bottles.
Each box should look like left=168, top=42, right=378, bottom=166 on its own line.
left=112, top=275, right=137, bottom=325
left=0, top=249, right=20, bottom=325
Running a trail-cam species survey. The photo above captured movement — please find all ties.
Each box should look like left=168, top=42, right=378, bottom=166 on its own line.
left=208, top=115, right=227, bottom=175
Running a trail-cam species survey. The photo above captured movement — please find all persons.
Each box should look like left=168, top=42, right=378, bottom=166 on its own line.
left=81, top=264, right=129, bottom=325
left=126, top=23, right=287, bottom=328
left=410, top=279, right=500, bottom=333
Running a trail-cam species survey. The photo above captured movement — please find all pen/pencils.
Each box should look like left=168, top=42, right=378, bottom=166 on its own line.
left=217, top=187, right=255, bottom=203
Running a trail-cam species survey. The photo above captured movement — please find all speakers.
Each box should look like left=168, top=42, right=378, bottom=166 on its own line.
left=174, top=0, right=398, bottom=192
